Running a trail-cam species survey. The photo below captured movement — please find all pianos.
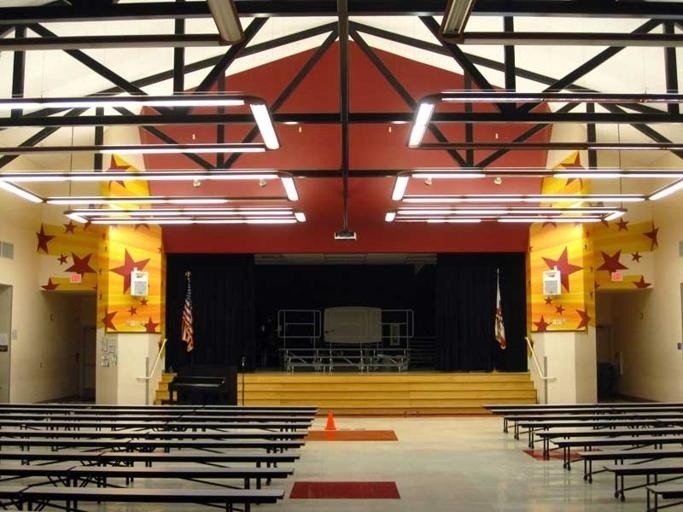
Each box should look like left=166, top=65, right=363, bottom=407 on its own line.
left=168, top=366, right=237, bottom=404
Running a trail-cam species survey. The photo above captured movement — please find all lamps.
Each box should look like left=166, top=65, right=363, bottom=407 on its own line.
left=385, top=92, right=682, bottom=224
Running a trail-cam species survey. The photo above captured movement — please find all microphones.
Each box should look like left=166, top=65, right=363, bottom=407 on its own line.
left=242, top=356, right=245, bottom=367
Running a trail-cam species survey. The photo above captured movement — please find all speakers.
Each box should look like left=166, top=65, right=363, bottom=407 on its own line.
left=544, top=269, right=562, bottom=298
left=131, top=272, right=148, bottom=296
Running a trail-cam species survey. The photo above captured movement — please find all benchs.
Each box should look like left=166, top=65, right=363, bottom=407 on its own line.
left=482, top=401, right=683, bottom=511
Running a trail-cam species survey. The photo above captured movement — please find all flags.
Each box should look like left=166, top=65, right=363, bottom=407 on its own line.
left=180, top=280, right=194, bottom=352
left=495, top=275, right=508, bottom=350
